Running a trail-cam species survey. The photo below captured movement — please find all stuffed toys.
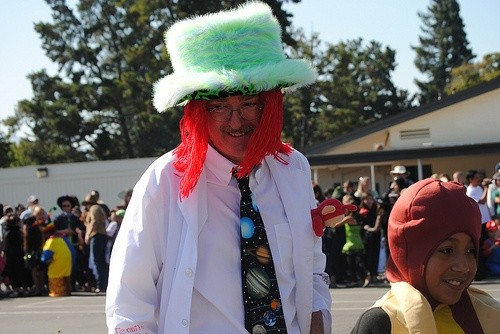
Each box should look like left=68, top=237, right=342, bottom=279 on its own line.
left=311, top=198, right=358, bottom=238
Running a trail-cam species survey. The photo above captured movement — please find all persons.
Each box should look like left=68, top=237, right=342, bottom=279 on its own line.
left=430, top=162, right=500, bottom=274
left=106, top=0, right=333, bottom=334
left=0, top=189, right=133, bottom=298
left=351, top=178, right=500, bottom=334
left=312, top=166, right=411, bottom=288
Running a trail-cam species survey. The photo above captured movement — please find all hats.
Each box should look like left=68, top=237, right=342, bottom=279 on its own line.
left=27, top=195, right=39, bottom=203
left=493, top=173, right=500, bottom=179
left=485, top=221, right=497, bottom=231
left=384, top=178, right=482, bottom=301
left=341, top=194, right=354, bottom=205
left=389, top=165, right=410, bottom=178
left=57, top=195, right=77, bottom=210
left=151, top=0, right=320, bottom=115
left=81, top=195, right=98, bottom=206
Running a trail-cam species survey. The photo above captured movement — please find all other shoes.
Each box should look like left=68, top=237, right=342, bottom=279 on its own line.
left=347, top=282, right=358, bottom=287
left=363, top=276, right=372, bottom=287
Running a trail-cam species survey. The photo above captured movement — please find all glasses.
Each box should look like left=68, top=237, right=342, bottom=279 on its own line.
left=62, top=204, right=70, bottom=208
left=209, top=103, right=264, bottom=123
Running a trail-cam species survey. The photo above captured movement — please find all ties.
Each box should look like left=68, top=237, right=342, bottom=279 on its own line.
left=231, top=168, right=288, bottom=334
